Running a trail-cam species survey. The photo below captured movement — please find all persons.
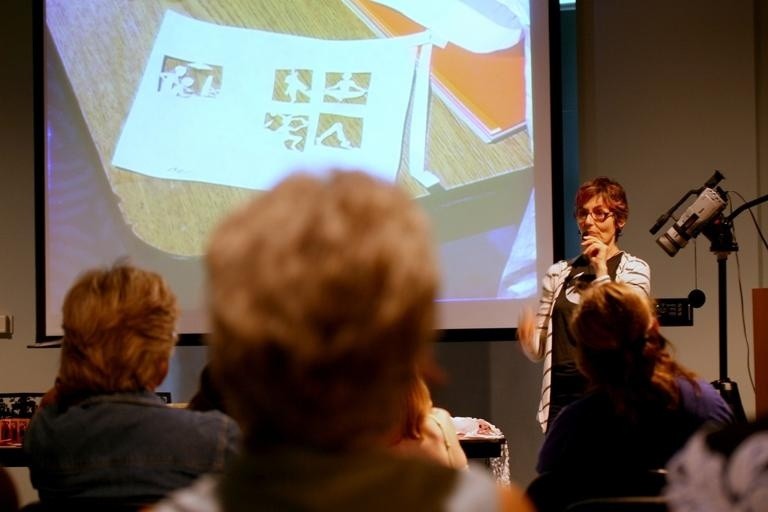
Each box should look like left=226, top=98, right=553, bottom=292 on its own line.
left=517, top=177, right=651, bottom=437
left=20, top=256, right=241, bottom=512
left=145, top=167, right=537, bottom=512
left=159, top=64, right=368, bottom=150
left=534, top=280, right=736, bottom=495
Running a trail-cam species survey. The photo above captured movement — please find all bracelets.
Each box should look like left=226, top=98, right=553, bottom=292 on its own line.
left=591, top=275, right=610, bottom=284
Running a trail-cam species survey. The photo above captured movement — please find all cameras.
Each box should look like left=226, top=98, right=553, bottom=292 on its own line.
left=656, top=187, right=728, bottom=256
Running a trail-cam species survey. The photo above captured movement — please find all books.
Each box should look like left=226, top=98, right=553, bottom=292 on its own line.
left=342, top=0, right=527, bottom=144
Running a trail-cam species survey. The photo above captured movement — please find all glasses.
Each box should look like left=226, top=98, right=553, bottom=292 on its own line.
left=573, top=208, right=614, bottom=223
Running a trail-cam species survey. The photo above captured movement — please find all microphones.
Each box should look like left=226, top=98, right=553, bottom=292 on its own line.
left=582, top=231, right=594, bottom=241
